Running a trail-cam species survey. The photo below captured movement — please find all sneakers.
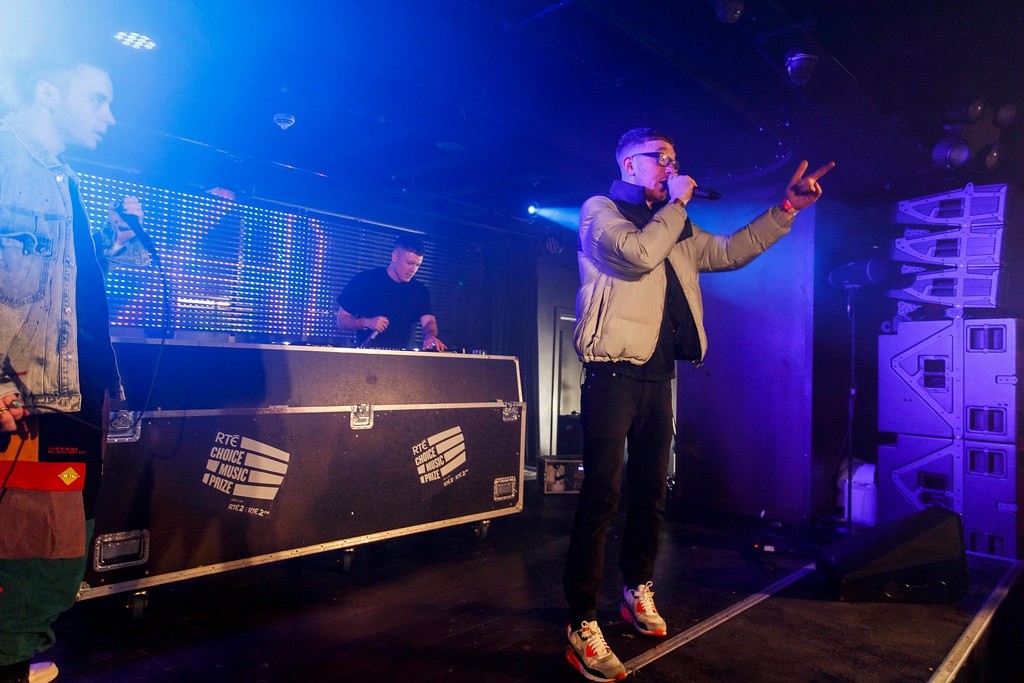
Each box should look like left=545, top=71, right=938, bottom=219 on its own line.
left=620, top=581, right=667, bottom=637
left=565, top=620, right=627, bottom=683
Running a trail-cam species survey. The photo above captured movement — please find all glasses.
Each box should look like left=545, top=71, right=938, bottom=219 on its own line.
left=631, top=152, right=680, bottom=173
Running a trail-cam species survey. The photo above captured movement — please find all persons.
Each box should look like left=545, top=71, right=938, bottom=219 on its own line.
left=567, top=127, right=837, bottom=683
left=335, top=233, right=448, bottom=354
left=0, top=37, right=145, bottom=683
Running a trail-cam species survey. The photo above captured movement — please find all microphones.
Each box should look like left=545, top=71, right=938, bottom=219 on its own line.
left=663, top=179, right=720, bottom=199
left=363, top=329, right=379, bottom=347
left=115, top=201, right=160, bottom=254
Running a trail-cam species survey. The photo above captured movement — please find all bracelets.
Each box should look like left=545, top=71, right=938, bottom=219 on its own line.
left=782, top=197, right=801, bottom=217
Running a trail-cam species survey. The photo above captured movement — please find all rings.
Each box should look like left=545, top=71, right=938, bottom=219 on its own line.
left=7, top=399, right=23, bottom=410
left=0, top=407, right=8, bottom=414
